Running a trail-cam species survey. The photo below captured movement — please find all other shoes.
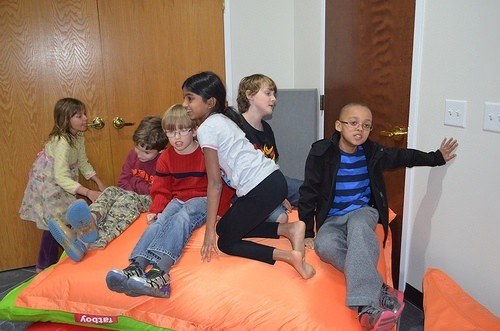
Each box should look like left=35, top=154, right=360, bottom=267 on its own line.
left=48, top=217, right=87, bottom=262
left=67, top=199, right=99, bottom=243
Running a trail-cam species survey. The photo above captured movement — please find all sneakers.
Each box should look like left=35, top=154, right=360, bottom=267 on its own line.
left=355, top=305, right=397, bottom=331
left=127, top=263, right=171, bottom=298
left=379, top=283, right=405, bottom=319
left=106, top=262, right=146, bottom=297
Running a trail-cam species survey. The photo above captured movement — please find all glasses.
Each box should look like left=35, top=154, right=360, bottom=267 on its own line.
left=166, top=128, right=192, bottom=137
left=340, top=121, right=373, bottom=131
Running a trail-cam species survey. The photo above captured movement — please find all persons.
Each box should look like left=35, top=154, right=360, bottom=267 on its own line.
left=236, top=74, right=305, bottom=224
left=299, top=102, right=458, bottom=331
left=181, top=72, right=316, bottom=281
left=19, top=97, right=107, bottom=273
left=48, top=115, right=170, bottom=262
left=106, top=102, right=236, bottom=298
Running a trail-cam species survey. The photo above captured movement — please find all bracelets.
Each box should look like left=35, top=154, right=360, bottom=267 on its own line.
left=86, top=189, right=91, bottom=197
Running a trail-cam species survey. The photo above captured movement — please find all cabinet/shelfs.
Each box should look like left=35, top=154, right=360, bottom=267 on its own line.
left=0, top=0, right=226, bottom=272
